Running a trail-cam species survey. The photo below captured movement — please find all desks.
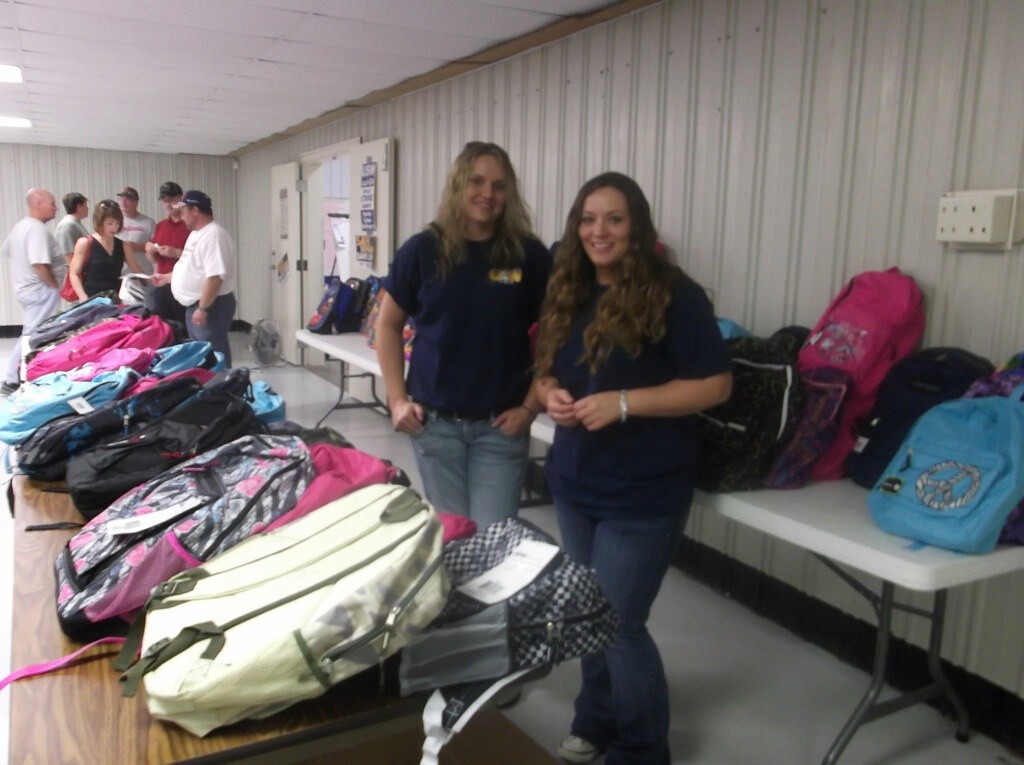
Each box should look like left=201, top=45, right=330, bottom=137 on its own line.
left=6, top=442, right=552, bottom=765
left=295, top=328, right=1024, bottom=765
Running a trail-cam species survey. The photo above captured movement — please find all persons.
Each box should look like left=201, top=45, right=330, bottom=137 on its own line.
left=374, top=142, right=554, bottom=710
left=535, top=171, right=732, bottom=765
left=145, top=181, right=194, bottom=341
left=112, top=187, right=157, bottom=311
left=69, top=199, right=145, bottom=310
left=0, top=189, right=64, bottom=395
left=170, top=190, right=236, bottom=374
left=53, top=193, right=91, bottom=323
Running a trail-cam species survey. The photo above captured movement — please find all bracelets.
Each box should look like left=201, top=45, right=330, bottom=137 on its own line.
left=619, top=388, right=628, bottom=423
left=198, top=305, right=209, bottom=312
left=522, top=405, right=539, bottom=416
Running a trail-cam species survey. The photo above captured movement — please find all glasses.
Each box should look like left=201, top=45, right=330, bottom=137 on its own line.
left=100, top=202, right=120, bottom=211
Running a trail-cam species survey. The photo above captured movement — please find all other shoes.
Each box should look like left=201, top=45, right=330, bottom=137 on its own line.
left=496, top=681, right=522, bottom=707
left=0, top=381, right=21, bottom=397
left=558, top=734, right=601, bottom=763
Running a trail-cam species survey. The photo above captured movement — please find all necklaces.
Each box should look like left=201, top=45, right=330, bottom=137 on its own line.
left=102, top=236, right=115, bottom=254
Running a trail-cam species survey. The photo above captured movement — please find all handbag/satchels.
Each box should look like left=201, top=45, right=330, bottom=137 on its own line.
left=59, top=233, right=94, bottom=303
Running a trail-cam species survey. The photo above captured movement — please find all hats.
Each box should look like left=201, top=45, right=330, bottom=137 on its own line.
left=117, top=187, right=138, bottom=200
left=157, top=181, right=183, bottom=198
left=172, top=190, right=212, bottom=208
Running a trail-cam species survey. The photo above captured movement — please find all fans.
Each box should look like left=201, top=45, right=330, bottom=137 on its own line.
left=249, top=318, right=288, bottom=373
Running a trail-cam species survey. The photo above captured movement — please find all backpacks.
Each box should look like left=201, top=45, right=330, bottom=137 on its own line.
left=0, top=274, right=616, bottom=765
left=711, top=266, right=1024, bottom=556
